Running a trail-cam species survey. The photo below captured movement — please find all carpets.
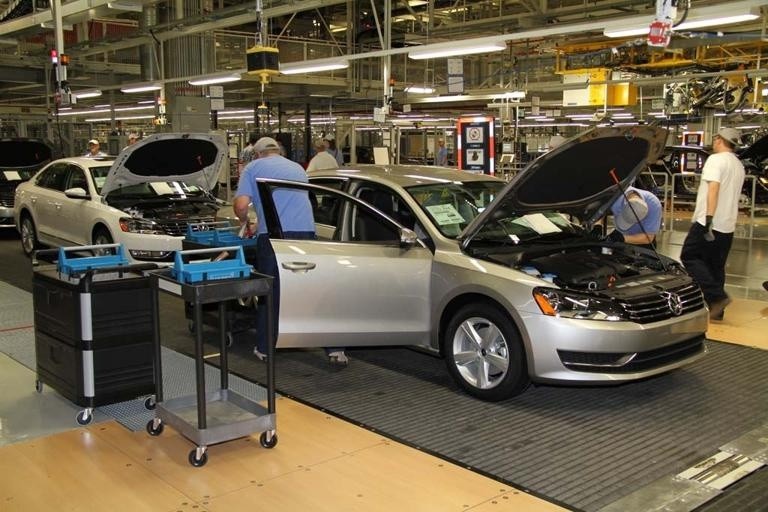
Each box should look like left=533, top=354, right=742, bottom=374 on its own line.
left=0, top=234, right=767, bottom=512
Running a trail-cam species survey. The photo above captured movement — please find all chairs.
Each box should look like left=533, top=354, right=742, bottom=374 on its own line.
left=354, top=189, right=400, bottom=242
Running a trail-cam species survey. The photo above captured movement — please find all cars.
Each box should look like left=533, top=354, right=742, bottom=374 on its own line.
left=337, top=143, right=427, bottom=166
left=250, top=124, right=712, bottom=406
left=1, top=137, right=53, bottom=230
left=634, top=133, right=767, bottom=210
left=14, top=131, right=254, bottom=282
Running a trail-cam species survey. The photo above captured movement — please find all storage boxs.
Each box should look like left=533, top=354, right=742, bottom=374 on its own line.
left=55, top=242, right=131, bottom=273
left=167, top=244, right=253, bottom=284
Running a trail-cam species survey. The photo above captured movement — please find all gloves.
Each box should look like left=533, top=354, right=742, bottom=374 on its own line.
left=703, top=214, right=715, bottom=243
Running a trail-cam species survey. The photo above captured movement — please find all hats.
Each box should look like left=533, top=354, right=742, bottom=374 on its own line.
left=89, top=139, right=100, bottom=145
left=252, top=137, right=280, bottom=152
left=614, top=197, right=648, bottom=233
left=323, top=134, right=336, bottom=141
left=129, top=134, right=140, bottom=140
left=712, top=127, right=740, bottom=146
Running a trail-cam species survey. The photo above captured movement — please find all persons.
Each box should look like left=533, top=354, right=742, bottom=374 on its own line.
left=122, top=132, right=141, bottom=151
left=304, top=138, right=339, bottom=173
left=322, top=135, right=343, bottom=166
left=84, top=139, right=107, bottom=157
left=583, top=185, right=663, bottom=249
left=288, top=142, right=316, bottom=162
left=436, top=137, right=448, bottom=165
left=414, top=193, right=462, bottom=239
left=278, top=140, right=287, bottom=158
left=233, top=136, right=348, bottom=367
left=680, top=126, right=746, bottom=325
left=238, top=132, right=259, bottom=175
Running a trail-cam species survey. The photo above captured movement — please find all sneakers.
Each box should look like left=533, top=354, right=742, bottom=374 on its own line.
left=711, top=311, right=725, bottom=324
left=707, top=296, right=732, bottom=317
left=328, top=351, right=350, bottom=366
left=253, top=345, right=273, bottom=363
